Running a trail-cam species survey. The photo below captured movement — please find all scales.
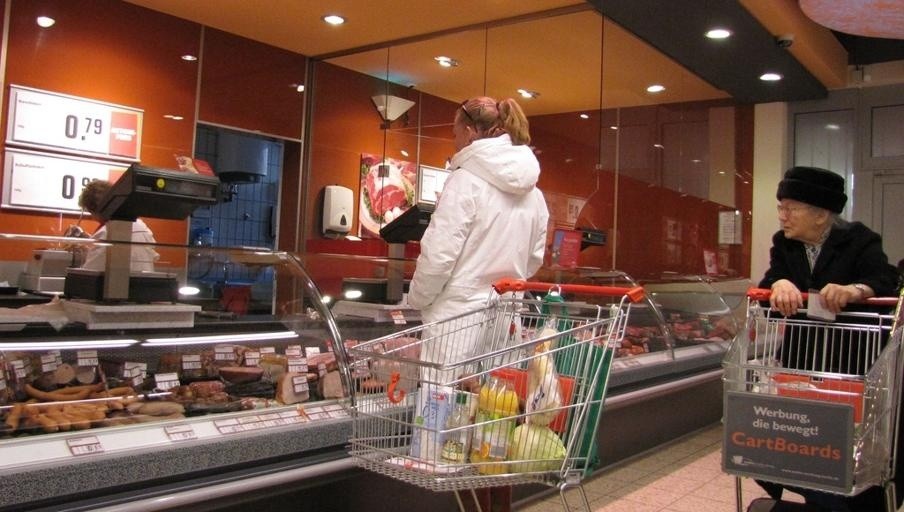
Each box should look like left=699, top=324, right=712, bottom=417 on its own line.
left=553, top=222, right=614, bottom=291
left=53, top=161, right=221, bottom=331
left=327, top=201, right=437, bottom=325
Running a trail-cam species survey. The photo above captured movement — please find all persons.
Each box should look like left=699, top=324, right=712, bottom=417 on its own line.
left=410, top=95, right=550, bottom=512
left=755, top=164, right=897, bottom=369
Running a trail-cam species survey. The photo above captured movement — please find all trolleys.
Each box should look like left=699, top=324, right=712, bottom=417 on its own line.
left=349, top=277, right=644, bottom=510
left=720, top=287, right=903, bottom=510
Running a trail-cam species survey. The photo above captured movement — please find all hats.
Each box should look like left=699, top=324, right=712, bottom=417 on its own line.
left=776, top=165, right=848, bottom=215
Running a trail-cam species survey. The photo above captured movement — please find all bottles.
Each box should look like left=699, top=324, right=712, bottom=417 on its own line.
left=470, top=370, right=498, bottom=465
left=481, top=373, right=518, bottom=475
left=443, top=393, right=471, bottom=463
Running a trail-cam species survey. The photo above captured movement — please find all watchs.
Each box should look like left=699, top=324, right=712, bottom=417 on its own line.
left=851, top=282, right=865, bottom=298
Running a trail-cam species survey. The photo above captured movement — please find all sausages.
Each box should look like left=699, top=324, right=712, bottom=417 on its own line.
left=6, top=404, right=23, bottom=431
left=139, top=403, right=186, bottom=415
left=64, top=406, right=107, bottom=425
left=74, top=403, right=109, bottom=412
left=45, top=406, right=72, bottom=431
left=59, top=405, right=91, bottom=430
left=24, top=383, right=91, bottom=401
left=133, top=413, right=186, bottom=422
left=49, top=382, right=105, bottom=395
left=91, top=401, right=125, bottom=410
left=25, top=406, right=40, bottom=428
left=109, top=387, right=135, bottom=395
left=125, top=402, right=144, bottom=413
left=33, top=413, right=60, bottom=433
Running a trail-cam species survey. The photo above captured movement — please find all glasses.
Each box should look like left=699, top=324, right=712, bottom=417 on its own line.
left=776, top=204, right=810, bottom=213
left=455, top=97, right=482, bottom=134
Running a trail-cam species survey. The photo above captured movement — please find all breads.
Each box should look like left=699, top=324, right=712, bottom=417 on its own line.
left=276, top=373, right=310, bottom=405
left=527, top=330, right=562, bottom=420
left=318, top=370, right=343, bottom=399
left=257, top=355, right=288, bottom=382
